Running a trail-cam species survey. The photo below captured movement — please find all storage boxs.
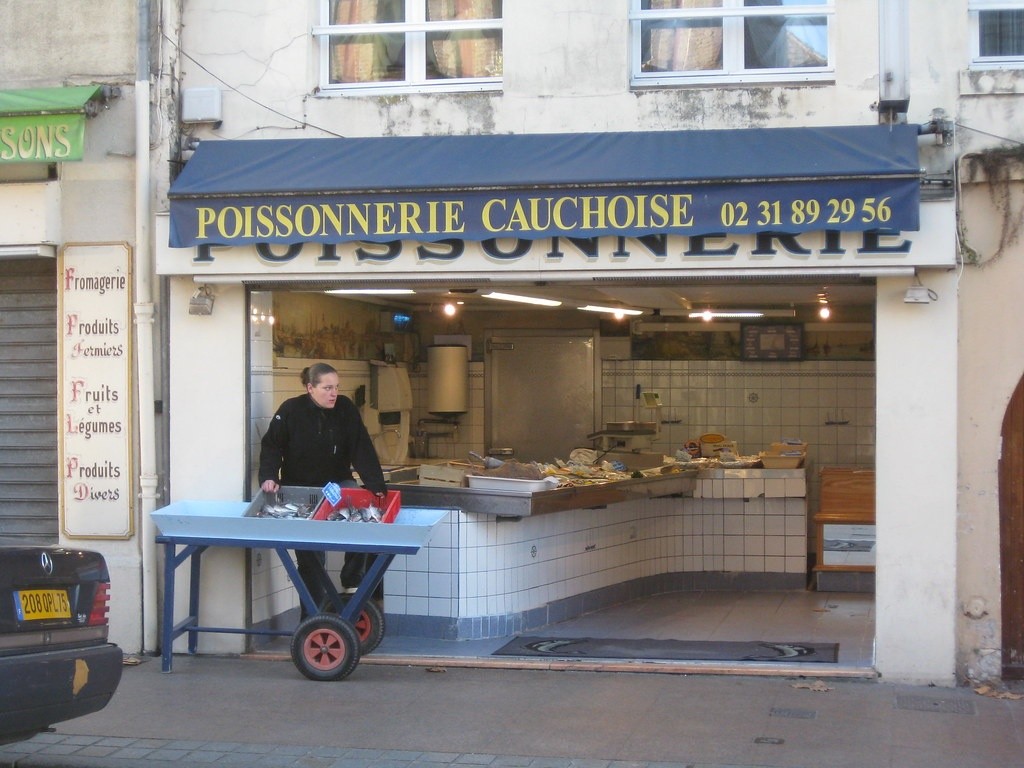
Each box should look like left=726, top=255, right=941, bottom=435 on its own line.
left=419, top=461, right=555, bottom=490
left=770, top=442, right=809, bottom=453
left=760, top=453, right=806, bottom=469
left=240, top=486, right=325, bottom=520
left=312, top=490, right=401, bottom=524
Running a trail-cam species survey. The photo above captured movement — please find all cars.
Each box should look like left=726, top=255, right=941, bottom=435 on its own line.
left=0, top=543, right=124, bottom=748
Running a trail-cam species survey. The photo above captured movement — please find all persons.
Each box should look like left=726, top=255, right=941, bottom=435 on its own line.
left=258, top=362, right=387, bottom=618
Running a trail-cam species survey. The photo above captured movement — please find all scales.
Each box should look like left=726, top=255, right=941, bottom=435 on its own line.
left=587, top=392, right=663, bottom=453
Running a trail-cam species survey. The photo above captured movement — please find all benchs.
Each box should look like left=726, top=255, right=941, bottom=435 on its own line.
left=811, top=466, right=876, bottom=572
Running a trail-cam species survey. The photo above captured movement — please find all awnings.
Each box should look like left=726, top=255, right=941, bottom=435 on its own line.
left=167, top=122, right=922, bottom=247
left=0, top=86, right=102, bottom=164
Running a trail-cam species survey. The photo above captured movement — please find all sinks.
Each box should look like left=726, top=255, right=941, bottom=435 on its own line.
left=350, top=466, right=419, bottom=485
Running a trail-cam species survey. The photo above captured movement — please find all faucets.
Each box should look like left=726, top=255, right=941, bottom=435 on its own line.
left=370, top=428, right=401, bottom=442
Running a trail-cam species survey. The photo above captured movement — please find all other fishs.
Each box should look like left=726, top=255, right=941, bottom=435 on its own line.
left=256, top=502, right=382, bottom=523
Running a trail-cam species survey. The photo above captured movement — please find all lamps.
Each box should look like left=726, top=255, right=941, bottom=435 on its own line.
left=660, top=309, right=796, bottom=317
left=188, top=282, right=214, bottom=315
left=449, top=288, right=654, bottom=317
left=903, top=272, right=939, bottom=304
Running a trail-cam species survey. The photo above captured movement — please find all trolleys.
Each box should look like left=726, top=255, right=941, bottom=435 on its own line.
left=146, top=498, right=452, bottom=682
